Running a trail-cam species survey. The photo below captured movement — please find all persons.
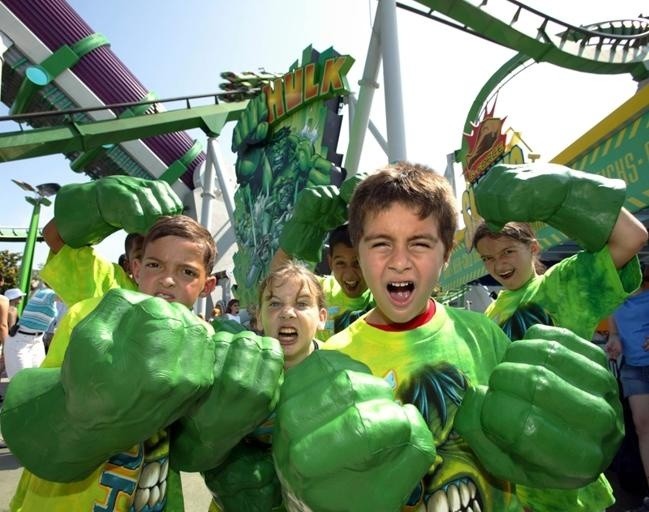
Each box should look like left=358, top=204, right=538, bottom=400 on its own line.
left=276, top=163, right=625, bottom=512
left=0, top=272, right=67, bottom=448
left=0, top=216, right=222, bottom=512
left=43, top=175, right=184, bottom=304
left=232, top=89, right=340, bottom=285
left=172, top=266, right=328, bottom=511
left=268, top=170, right=378, bottom=345
left=475, top=164, right=647, bottom=512
left=199, top=298, right=261, bottom=334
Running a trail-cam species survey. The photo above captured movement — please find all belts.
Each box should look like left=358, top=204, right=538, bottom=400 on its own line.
left=17, top=330, right=44, bottom=335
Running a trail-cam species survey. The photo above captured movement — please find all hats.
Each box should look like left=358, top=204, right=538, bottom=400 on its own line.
left=4, top=288, right=27, bottom=300
